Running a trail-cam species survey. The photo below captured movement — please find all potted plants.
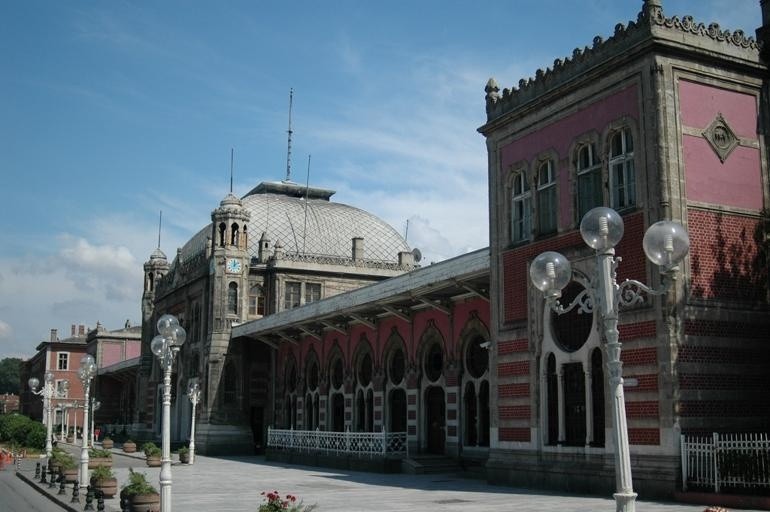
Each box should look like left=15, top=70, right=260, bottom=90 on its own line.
left=48, top=436, right=195, bottom=512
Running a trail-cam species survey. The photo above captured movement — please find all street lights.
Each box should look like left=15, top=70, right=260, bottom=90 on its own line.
left=27, top=373, right=72, bottom=476
left=75, top=352, right=99, bottom=491
left=150, top=311, right=185, bottom=511
left=40, top=398, right=102, bottom=449
left=529, top=205, right=698, bottom=511
